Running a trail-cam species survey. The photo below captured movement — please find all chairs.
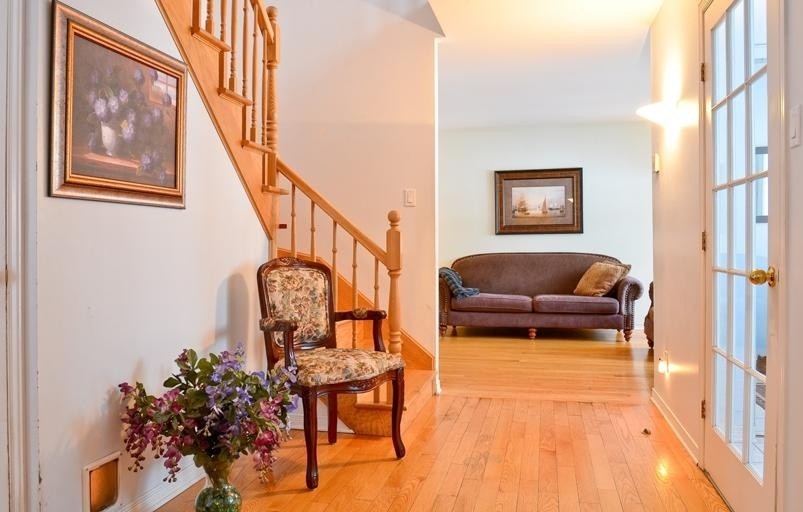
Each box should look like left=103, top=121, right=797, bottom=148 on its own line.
left=256, top=255, right=407, bottom=490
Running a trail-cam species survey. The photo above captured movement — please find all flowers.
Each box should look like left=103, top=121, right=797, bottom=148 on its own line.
left=118, top=344, right=299, bottom=484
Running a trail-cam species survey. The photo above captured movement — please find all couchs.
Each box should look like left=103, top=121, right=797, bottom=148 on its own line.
left=438, top=251, right=645, bottom=342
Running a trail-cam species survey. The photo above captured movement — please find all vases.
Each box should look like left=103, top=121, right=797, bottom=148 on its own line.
left=193, top=459, right=242, bottom=512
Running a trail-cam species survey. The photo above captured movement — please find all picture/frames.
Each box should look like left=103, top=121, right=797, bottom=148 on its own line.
left=46, top=0, right=190, bottom=211
left=494, top=166, right=584, bottom=235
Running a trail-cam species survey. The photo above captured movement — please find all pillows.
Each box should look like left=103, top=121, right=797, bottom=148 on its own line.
left=573, top=262, right=626, bottom=297
